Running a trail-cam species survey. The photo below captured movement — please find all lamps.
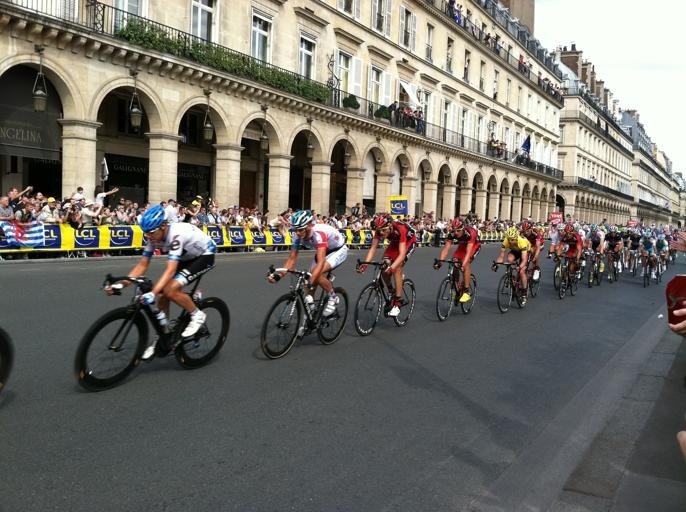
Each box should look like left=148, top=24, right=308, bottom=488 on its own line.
left=344, top=133, right=351, bottom=168
left=376, top=143, right=383, bottom=174
left=202, top=96, right=214, bottom=143
left=306, top=125, right=315, bottom=161
left=444, top=159, right=670, bottom=225
left=260, top=113, right=269, bottom=152
left=425, top=154, right=431, bottom=181
left=130, top=74, right=143, bottom=132
left=33, top=51, right=48, bottom=112
left=402, top=146, right=408, bottom=176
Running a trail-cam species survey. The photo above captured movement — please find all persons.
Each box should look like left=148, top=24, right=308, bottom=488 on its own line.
left=433, top=219, right=482, bottom=302
left=268, top=210, right=347, bottom=336
left=546, top=224, right=569, bottom=259
left=535, top=222, right=551, bottom=240
left=522, top=151, right=527, bottom=165
left=553, top=224, right=584, bottom=279
left=389, top=101, right=424, bottom=134
left=160, top=196, right=295, bottom=254
left=667, top=308, right=684, bottom=455
left=1, top=185, right=150, bottom=260
left=395, top=214, right=453, bottom=248
left=519, top=220, right=544, bottom=280
left=572, top=222, right=684, bottom=278
left=448, top=1, right=566, bottom=104
left=356, top=212, right=416, bottom=316
left=105, top=205, right=217, bottom=359
left=491, top=226, right=532, bottom=304
left=311, top=203, right=382, bottom=249
left=465, top=215, right=516, bottom=244
left=490, top=139, right=507, bottom=155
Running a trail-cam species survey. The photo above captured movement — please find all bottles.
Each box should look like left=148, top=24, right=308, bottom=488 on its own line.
left=48, top=197, right=55, bottom=203
left=85, top=200, right=93, bottom=208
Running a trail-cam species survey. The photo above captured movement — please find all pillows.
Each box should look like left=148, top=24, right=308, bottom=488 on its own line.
left=371, top=213, right=392, bottom=230
left=558, top=221, right=677, bottom=240
left=291, top=210, right=312, bottom=229
left=140, top=205, right=166, bottom=233
left=505, top=226, right=518, bottom=240
left=452, top=217, right=465, bottom=230
left=522, top=222, right=531, bottom=231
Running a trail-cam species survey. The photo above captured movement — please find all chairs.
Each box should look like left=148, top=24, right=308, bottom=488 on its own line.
left=323, top=295, right=339, bottom=316
left=533, top=270, right=539, bottom=280
left=181, top=313, right=206, bottom=337
left=459, top=293, right=471, bottom=302
left=608, top=253, right=678, bottom=280
left=521, top=295, right=528, bottom=304
left=388, top=305, right=400, bottom=316
left=142, top=346, right=155, bottom=359
left=599, top=262, right=604, bottom=272
left=581, top=260, right=585, bottom=266
left=576, top=268, right=581, bottom=279
left=298, top=327, right=307, bottom=336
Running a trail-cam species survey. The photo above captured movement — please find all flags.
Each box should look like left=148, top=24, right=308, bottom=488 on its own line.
left=521, top=134, right=530, bottom=155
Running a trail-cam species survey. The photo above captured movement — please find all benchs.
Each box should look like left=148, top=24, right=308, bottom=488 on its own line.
left=521, top=134, right=530, bottom=155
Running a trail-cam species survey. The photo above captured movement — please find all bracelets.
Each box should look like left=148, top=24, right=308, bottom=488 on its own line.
left=279, top=272, right=285, bottom=277
